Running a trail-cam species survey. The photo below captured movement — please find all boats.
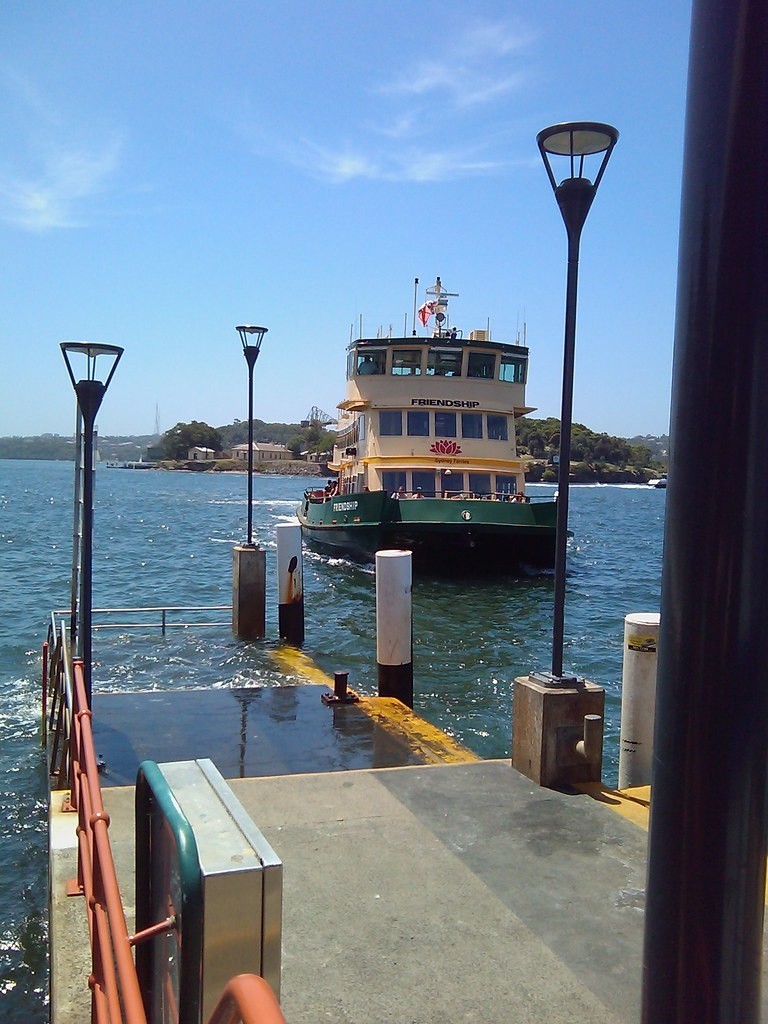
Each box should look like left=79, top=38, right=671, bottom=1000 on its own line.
left=293, top=272, right=576, bottom=584
left=104, top=452, right=160, bottom=470
left=646, top=472, right=668, bottom=488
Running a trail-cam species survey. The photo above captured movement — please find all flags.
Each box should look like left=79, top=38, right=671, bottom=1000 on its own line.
left=418, top=300, right=436, bottom=326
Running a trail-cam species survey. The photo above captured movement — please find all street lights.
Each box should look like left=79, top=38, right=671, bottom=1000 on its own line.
left=60, top=339, right=126, bottom=711
left=535, top=119, right=621, bottom=680
left=236, top=325, right=269, bottom=545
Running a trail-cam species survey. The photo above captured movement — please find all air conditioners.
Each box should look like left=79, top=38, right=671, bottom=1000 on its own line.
left=469, top=329, right=488, bottom=341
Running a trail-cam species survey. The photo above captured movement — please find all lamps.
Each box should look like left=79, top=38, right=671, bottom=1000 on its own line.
left=436, top=312, right=444, bottom=322
left=444, top=469, right=451, bottom=474
left=451, top=326, right=457, bottom=334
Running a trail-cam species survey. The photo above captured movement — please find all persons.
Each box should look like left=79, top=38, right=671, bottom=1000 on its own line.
left=434, top=367, right=445, bottom=376
left=450, top=327, right=457, bottom=339
left=391, top=486, right=408, bottom=499
left=357, top=355, right=377, bottom=375
left=323, top=480, right=340, bottom=500
left=459, top=489, right=530, bottom=503
left=411, top=486, right=425, bottom=499
left=362, top=485, right=370, bottom=492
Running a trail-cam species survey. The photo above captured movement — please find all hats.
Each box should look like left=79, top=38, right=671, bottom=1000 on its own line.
left=416, top=487, right=421, bottom=490
